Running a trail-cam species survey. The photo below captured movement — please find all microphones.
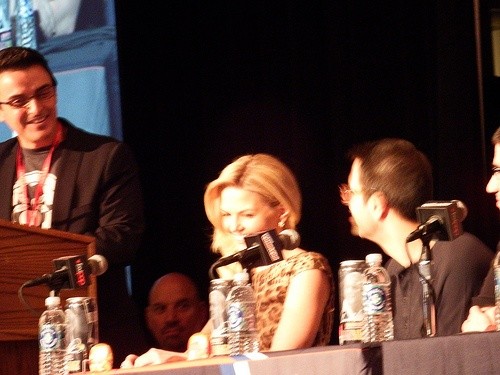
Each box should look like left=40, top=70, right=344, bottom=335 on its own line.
left=406, top=200, right=467, bottom=244
left=211, top=228, right=300, bottom=271
left=24, top=254, right=108, bottom=289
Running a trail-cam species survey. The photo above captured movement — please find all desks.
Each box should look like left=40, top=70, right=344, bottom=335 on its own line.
left=113, top=331, right=500, bottom=375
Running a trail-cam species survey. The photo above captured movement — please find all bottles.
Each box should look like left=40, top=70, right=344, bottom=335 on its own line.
left=38, top=296, right=64, bottom=375
left=226, top=272, right=259, bottom=358
left=493, top=252, right=500, bottom=332
left=361, top=253, right=395, bottom=343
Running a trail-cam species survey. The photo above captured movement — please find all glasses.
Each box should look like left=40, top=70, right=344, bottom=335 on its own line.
left=0, top=86, right=55, bottom=107
left=340, top=186, right=377, bottom=201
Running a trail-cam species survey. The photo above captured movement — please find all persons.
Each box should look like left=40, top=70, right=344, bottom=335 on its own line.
left=121, top=153, right=336, bottom=369
left=0, top=46, right=142, bottom=300
left=461, top=127, right=500, bottom=332
left=145, top=273, right=210, bottom=352
left=339, top=139, right=493, bottom=342
